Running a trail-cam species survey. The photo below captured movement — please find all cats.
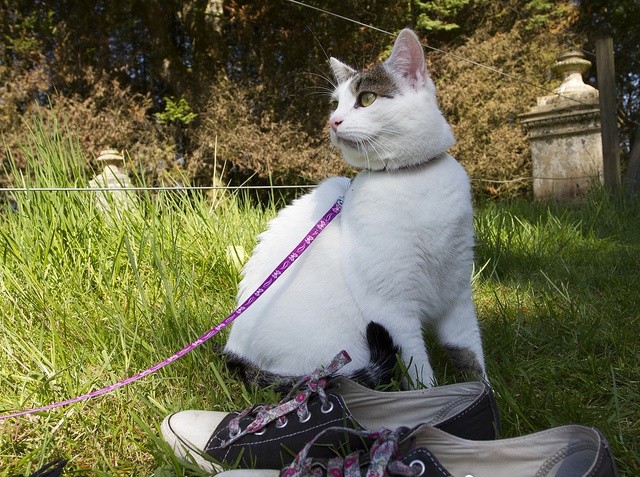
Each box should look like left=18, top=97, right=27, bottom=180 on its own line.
left=219, top=28, right=498, bottom=395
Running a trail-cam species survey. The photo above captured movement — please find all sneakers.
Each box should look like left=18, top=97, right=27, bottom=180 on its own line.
left=161, top=350, right=502, bottom=474
left=209, top=424, right=617, bottom=477
